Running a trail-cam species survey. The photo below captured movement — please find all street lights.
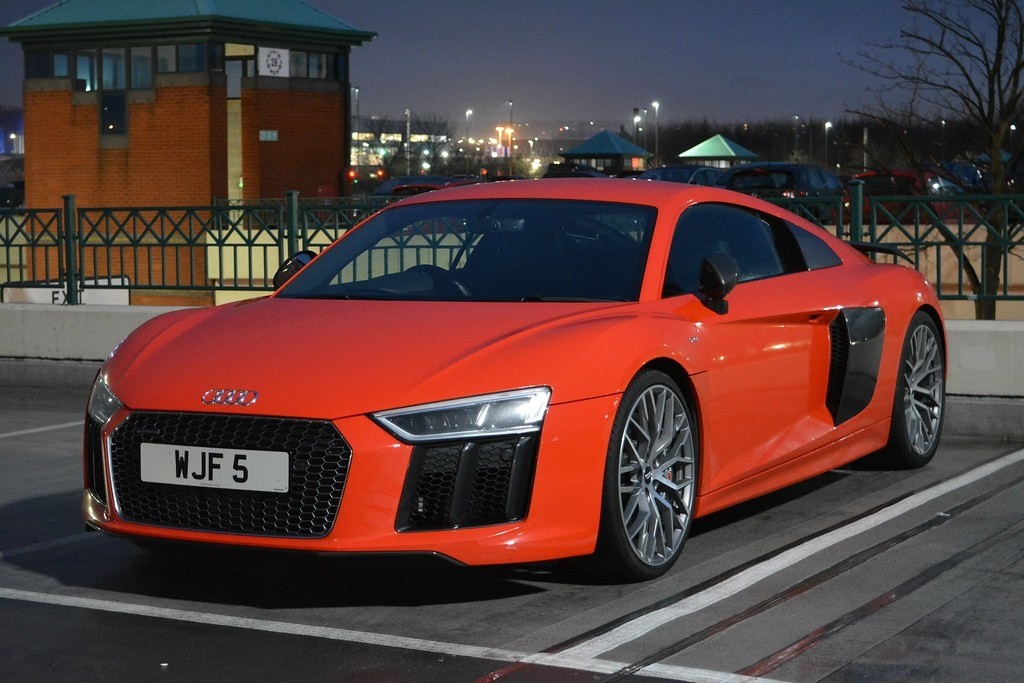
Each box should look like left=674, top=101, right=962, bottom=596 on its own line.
left=496, top=125, right=504, bottom=156
left=505, top=127, right=515, bottom=178
left=351, top=85, right=362, bottom=166
left=405, top=109, right=413, bottom=174
left=507, top=100, right=513, bottom=177
left=633, top=115, right=640, bottom=144
left=825, top=121, right=833, bottom=167
left=464, top=109, right=473, bottom=173
left=651, top=101, right=659, bottom=168
left=794, top=113, right=800, bottom=150
left=642, top=108, right=647, bottom=148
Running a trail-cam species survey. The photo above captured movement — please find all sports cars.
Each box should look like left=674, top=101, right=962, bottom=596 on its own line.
left=77, top=174, right=950, bottom=583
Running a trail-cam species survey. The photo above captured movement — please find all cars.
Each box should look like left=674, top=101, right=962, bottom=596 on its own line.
left=385, top=181, right=480, bottom=238
left=828, top=170, right=985, bottom=226
left=368, top=173, right=474, bottom=214
left=638, top=166, right=727, bottom=188
left=924, top=157, right=1023, bottom=221
left=714, top=162, right=840, bottom=226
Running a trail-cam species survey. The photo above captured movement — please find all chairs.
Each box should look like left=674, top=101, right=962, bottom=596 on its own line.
left=463, top=232, right=528, bottom=296
